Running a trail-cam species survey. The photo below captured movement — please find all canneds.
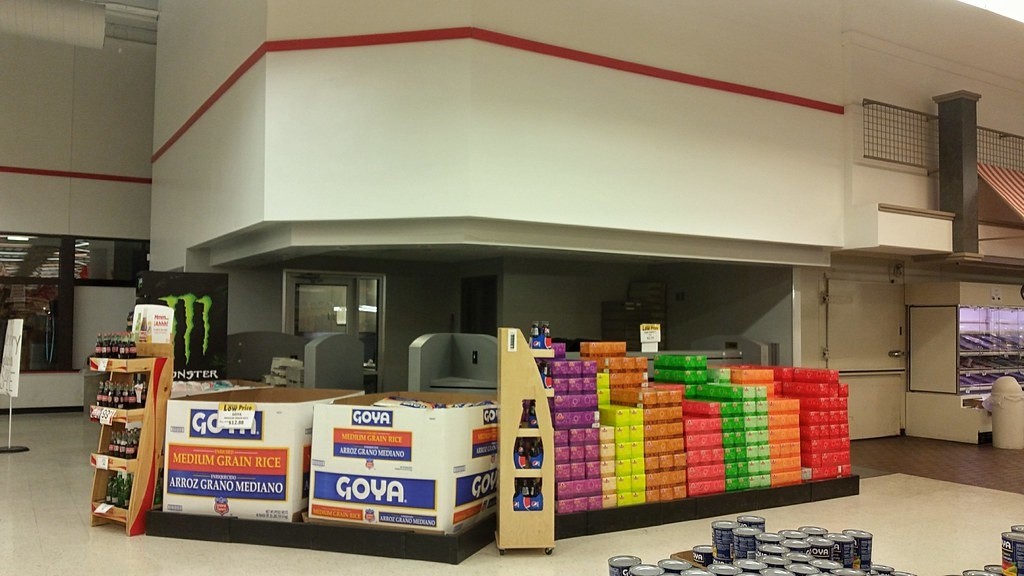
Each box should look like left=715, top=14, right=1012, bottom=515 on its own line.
left=608, top=514, right=1024, bottom=576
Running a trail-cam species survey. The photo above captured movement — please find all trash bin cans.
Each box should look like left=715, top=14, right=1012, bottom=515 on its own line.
left=991, top=376, right=1024, bottom=450
left=79, top=368, right=111, bottom=418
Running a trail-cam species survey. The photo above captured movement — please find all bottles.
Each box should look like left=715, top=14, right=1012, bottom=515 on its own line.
left=513, top=399, right=544, bottom=511
left=96, top=331, right=164, bottom=508
left=528, top=320, right=552, bottom=389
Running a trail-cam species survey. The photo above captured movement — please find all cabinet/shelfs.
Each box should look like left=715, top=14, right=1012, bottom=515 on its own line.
left=88, top=342, right=175, bottom=537
left=494, top=326, right=555, bottom=556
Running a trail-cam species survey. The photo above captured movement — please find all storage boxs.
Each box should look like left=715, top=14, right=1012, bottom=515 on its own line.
left=160, top=325, right=851, bottom=537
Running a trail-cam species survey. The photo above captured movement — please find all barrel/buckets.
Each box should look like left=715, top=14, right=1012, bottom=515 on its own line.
left=607, top=517, right=1024, bottom=576
